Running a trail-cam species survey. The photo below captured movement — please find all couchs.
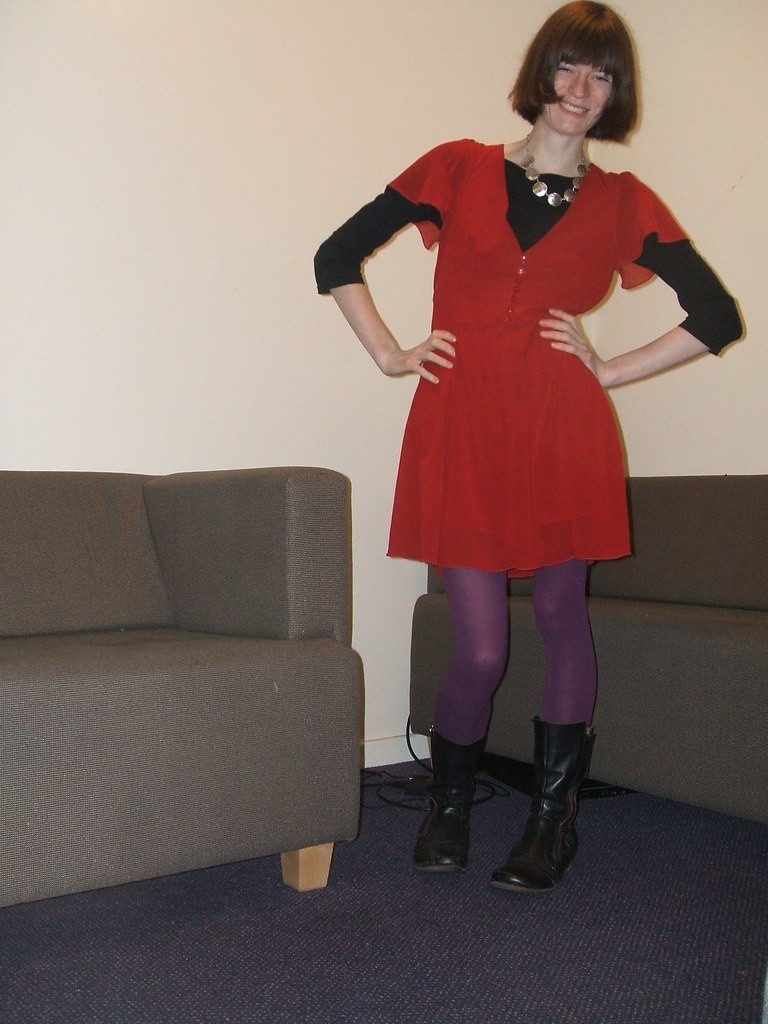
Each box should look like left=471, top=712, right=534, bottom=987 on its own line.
left=409, top=473, right=767, bottom=821
left=0, top=466, right=367, bottom=915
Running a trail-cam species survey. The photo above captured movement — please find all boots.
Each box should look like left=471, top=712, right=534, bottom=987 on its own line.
left=413, top=722, right=488, bottom=872
left=489, top=715, right=596, bottom=895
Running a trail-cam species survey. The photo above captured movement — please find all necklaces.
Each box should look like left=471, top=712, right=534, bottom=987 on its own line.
left=522, top=134, right=586, bottom=207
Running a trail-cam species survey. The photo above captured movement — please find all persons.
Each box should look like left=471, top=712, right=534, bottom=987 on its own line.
left=314, top=1, right=743, bottom=895
